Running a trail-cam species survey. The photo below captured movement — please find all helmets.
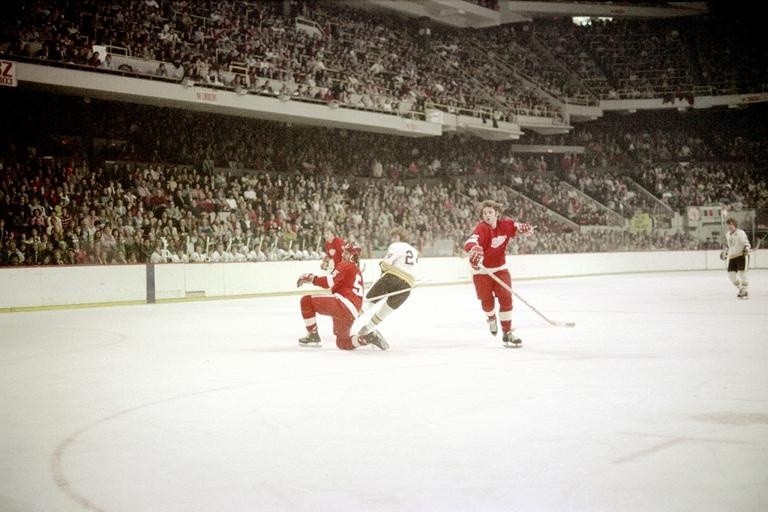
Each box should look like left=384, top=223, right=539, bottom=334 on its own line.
left=341, top=241, right=361, bottom=256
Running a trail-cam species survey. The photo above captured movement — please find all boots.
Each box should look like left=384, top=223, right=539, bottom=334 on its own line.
left=489, top=317, right=498, bottom=335
left=299, top=332, right=321, bottom=343
left=503, top=332, right=522, bottom=344
left=737, top=289, right=748, bottom=296
left=364, top=331, right=387, bottom=351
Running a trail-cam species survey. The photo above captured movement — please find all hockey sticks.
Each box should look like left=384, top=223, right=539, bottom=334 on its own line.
left=724, top=239, right=760, bottom=259
left=478, top=263, right=576, bottom=329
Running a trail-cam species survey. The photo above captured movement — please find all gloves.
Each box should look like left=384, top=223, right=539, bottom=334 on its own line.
left=518, top=223, right=532, bottom=233
left=297, top=273, right=317, bottom=288
left=468, top=247, right=483, bottom=271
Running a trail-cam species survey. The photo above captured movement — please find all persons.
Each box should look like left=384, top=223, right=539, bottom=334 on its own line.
left=716, top=218, right=752, bottom=298
left=464, top=200, right=535, bottom=345
left=0, top=1, right=768, bottom=270
left=295, top=242, right=389, bottom=354
left=355, top=228, right=422, bottom=335
